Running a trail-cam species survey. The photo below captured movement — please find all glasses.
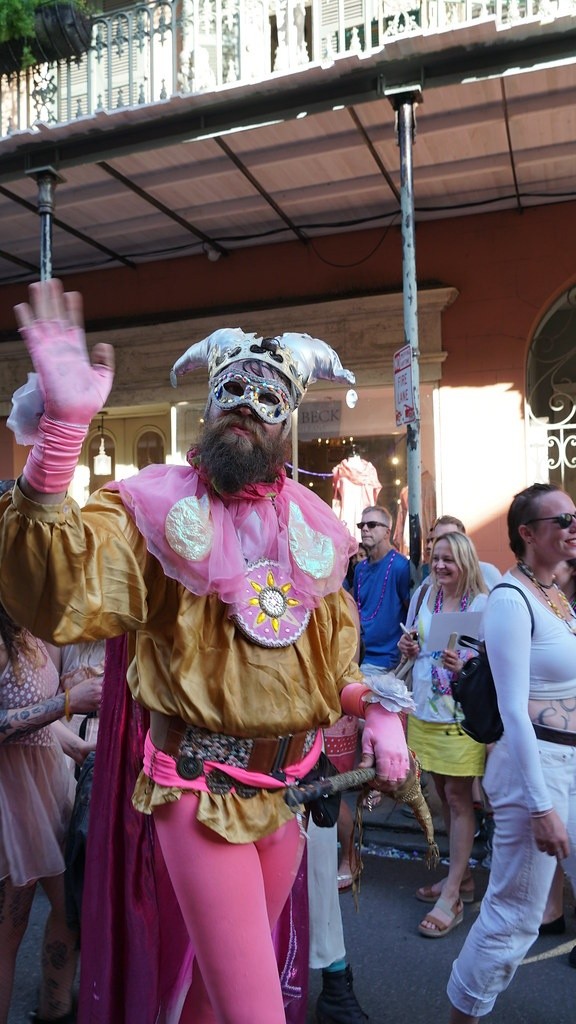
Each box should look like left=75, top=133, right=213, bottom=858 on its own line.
left=524, top=513, right=576, bottom=529
left=357, top=521, right=385, bottom=529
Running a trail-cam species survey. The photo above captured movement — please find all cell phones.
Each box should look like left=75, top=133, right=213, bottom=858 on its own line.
left=400, top=623, right=413, bottom=643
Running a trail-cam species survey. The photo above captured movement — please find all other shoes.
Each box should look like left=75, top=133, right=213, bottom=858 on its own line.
left=538, top=913, right=567, bottom=936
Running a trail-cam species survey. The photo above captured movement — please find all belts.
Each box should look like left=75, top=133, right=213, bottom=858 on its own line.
left=533, top=724, right=576, bottom=747
left=148, top=708, right=316, bottom=775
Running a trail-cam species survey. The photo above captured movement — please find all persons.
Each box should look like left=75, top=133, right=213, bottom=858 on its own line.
left=444, top=483, right=576, bottom=1023
left=13, top=277, right=412, bottom=1024
left=1, top=479, right=98, bottom=1024
left=306, top=505, right=574, bottom=1024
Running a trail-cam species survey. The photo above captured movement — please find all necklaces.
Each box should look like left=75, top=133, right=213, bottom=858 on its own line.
left=357, top=551, right=396, bottom=622
left=430, top=586, right=473, bottom=696
left=518, top=560, right=576, bottom=637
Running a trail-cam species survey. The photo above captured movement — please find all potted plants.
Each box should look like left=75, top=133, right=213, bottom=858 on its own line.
left=0, top=0, right=104, bottom=126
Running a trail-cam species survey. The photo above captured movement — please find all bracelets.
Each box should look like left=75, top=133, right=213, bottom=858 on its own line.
left=531, top=806, right=556, bottom=818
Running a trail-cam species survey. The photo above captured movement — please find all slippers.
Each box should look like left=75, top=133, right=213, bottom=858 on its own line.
left=336, top=859, right=364, bottom=895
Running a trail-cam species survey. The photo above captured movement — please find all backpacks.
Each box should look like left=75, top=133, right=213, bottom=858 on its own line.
left=447, top=581, right=538, bottom=745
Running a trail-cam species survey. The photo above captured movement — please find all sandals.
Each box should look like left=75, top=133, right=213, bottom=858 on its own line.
left=415, top=872, right=475, bottom=904
left=418, top=895, right=464, bottom=937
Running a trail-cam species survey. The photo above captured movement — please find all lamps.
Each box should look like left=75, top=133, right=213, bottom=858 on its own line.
left=94, top=410, right=112, bottom=477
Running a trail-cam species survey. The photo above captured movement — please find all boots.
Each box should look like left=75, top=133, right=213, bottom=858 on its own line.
left=314, top=962, right=370, bottom=1024
